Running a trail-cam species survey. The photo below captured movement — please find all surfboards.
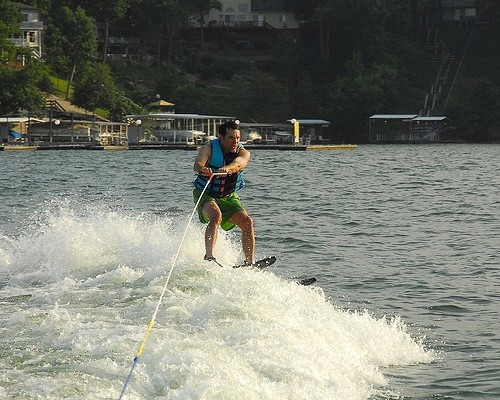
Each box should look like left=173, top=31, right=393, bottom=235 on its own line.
left=230, top=255, right=316, bottom=286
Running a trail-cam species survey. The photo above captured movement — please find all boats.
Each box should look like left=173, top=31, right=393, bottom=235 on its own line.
left=153, top=130, right=205, bottom=142
left=274, top=130, right=292, bottom=145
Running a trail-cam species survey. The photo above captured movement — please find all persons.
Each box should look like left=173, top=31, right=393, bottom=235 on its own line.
left=192, top=121, right=255, bottom=266
